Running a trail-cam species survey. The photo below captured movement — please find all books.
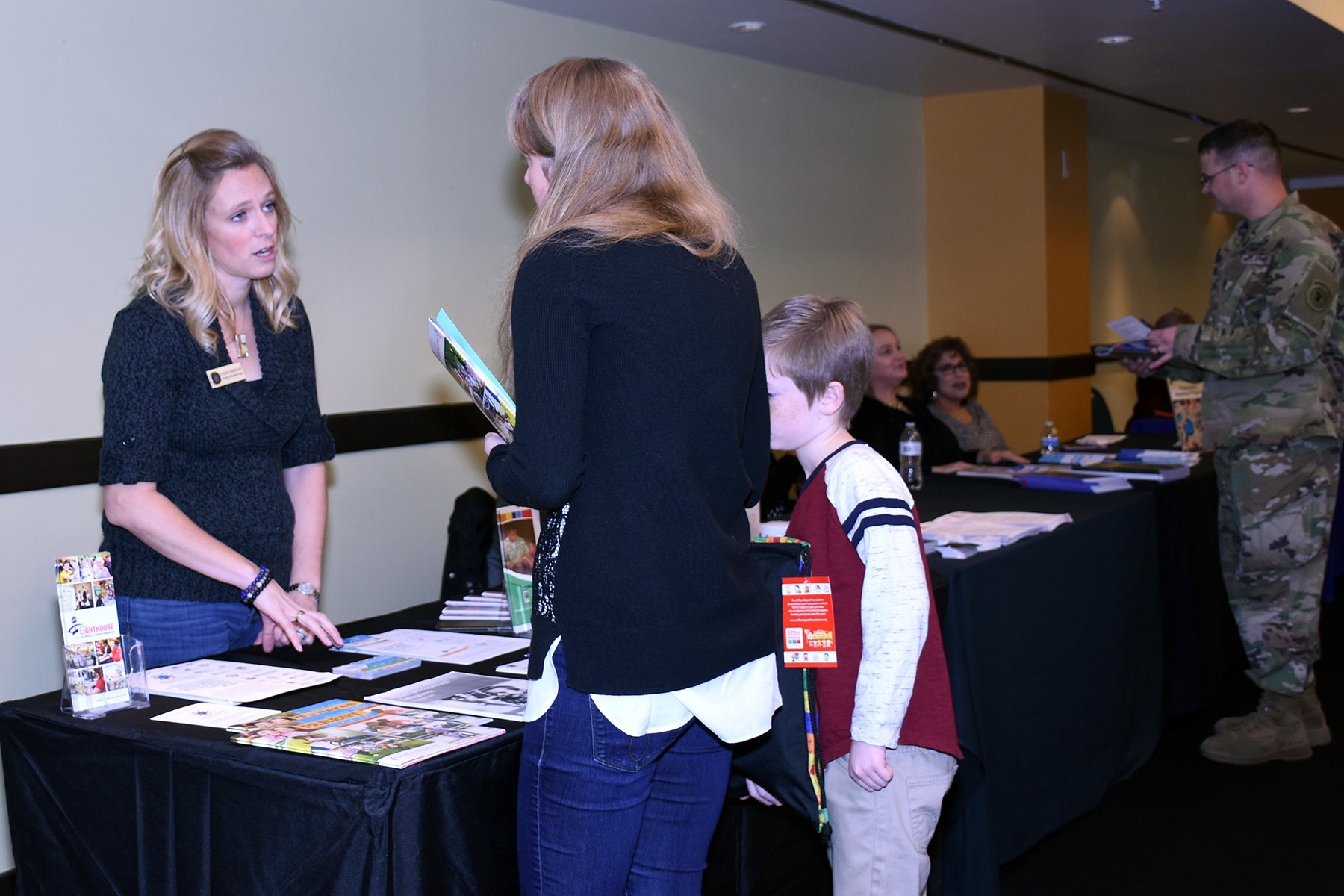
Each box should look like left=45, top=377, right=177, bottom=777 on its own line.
left=958, top=450, right=1202, bottom=493
left=426, top=308, right=518, bottom=445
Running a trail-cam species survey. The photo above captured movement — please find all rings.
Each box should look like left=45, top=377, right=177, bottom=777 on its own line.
left=296, top=630, right=306, bottom=639
left=296, top=610, right=304, bottom=622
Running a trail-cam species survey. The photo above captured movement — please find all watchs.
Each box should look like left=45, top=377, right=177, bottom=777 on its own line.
left=285, top=581, right=320, bottom=601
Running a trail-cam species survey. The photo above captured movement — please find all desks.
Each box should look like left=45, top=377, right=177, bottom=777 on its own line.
left=0, top=433, right=1250, bottom=896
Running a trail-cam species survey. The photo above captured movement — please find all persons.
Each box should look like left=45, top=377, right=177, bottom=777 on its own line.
left=93, top=130, right=344, bottom=670
left=447, top=348, right=516, bottom=440
left=759, top=295, right=962, bottom=896
left=248, top=709, right=481, bottom=753
left=503, top=524, right=529, bottom=569
left=1127, top=307, right=1197, bottom=434
left=61, top=557, right=125, bottom=695
left=1121, top=121, right=1344, bottom=765
left=849, top=324, right=1031, bottom=473
left=486, top=59, right=782, bottom=896
left=909, top=337, right=1010, bottom=451
left=486, top=686, right=528, bottom=702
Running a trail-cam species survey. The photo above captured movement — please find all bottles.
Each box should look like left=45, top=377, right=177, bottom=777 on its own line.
left=1040, top=421, right=1059, bottom=456
left=898, top=422, right=923, bottom=491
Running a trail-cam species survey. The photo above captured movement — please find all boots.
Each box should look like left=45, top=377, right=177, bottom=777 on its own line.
left=1201, top=693, right=1314, bottom=765
left=1216, top=684, right=1332, bottom=746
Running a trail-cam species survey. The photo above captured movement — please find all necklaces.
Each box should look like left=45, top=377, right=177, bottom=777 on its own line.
left=235, top=333, right=249, bottom=358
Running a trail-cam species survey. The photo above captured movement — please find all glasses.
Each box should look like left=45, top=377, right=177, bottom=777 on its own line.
left=932, top=360, right=971, bottom=376
left=1203, top=163, right=1255, bottom=190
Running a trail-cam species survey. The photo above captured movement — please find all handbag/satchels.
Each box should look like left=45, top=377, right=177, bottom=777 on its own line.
left=746, top=536, right=828, bottom=830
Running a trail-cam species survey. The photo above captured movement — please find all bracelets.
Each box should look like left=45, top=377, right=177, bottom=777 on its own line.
left=239, top=566, right=272, bottom=606
